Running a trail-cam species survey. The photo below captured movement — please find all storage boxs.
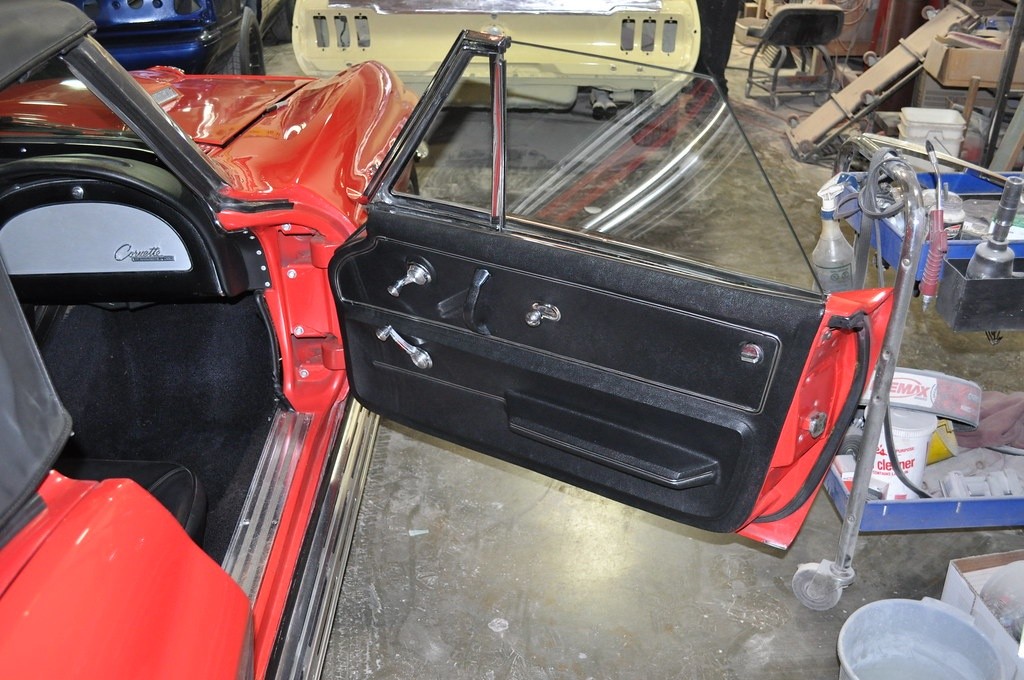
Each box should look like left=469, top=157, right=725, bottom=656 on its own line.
left=923, top=34, right=1023, bottom=92
left=942, top=550, right=1024, bottom=680
left=736, top=17, right=768, bottom=46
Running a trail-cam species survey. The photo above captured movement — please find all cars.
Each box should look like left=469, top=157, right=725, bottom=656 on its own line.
left=291, top=0, right=701, bottom=119
left=67, top=1, right=294, bottom=74
left=0, top=0, right=896, bottom=680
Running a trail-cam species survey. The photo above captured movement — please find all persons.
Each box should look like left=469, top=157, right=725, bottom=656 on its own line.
left=682, top=0, right=741, bottom=112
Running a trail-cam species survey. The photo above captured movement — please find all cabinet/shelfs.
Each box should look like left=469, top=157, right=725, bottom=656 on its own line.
left=794, top=170, right=1024, bottom=612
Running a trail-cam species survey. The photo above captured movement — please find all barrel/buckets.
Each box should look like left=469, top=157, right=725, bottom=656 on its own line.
left=897, top=106, right=968, bottom=173
left=837, top=595, right=1007, bottom=680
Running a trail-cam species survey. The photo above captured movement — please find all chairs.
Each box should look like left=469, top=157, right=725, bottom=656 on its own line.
left=57, top=458, right=211, bottom=545
left=744, top=2, right=845, bottom=111
